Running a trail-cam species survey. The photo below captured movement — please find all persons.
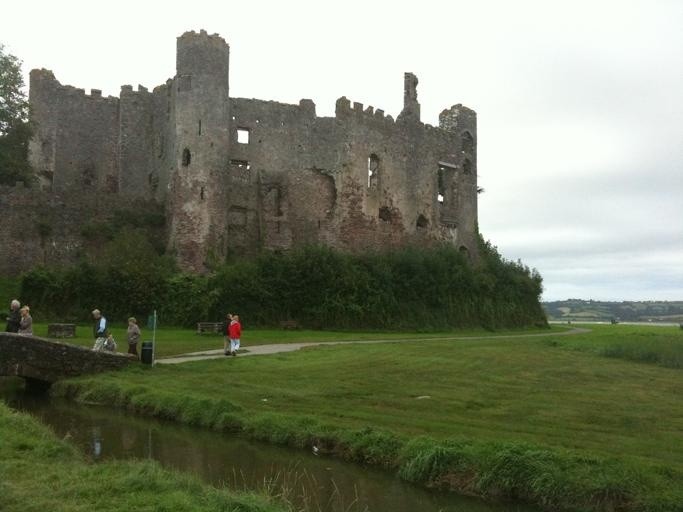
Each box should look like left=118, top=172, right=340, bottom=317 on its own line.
left=226, top=314, right=241, bottom=356
left=125, top=317, right=140, bottom=361
left=89, top=309, right=106, bottom=352
left=18, top=306, right=32, bottom=336
left=4, top=299, right=20, bottom=332
left=102, top=335, right=115, bottom=351
left=221, top=313, right=233, bottom=355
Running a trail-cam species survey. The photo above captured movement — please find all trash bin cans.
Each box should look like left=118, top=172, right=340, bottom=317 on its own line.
left=142, top=341, right=153, bottom=364
